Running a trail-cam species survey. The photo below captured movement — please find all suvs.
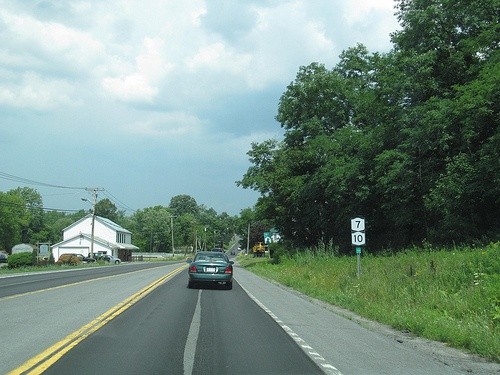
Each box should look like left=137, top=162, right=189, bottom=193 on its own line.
left=57, top=253, right=95, bottom=266
left=211, top=248, right=225, bottom=253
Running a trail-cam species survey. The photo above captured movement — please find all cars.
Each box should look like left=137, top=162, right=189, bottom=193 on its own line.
left=186, top=251, right=235, bottom=290
left=0, top=251, right=10, bottom=263
left=88, top=253, right=121, bottom=265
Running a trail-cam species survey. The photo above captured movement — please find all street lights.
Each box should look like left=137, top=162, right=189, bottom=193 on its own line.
left=80, top=197, right=95, bottom=253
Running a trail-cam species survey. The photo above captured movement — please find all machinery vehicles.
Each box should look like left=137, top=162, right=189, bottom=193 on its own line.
left=253, top=241, right=269, bottom=258
